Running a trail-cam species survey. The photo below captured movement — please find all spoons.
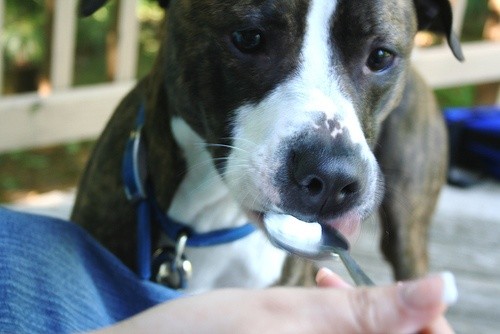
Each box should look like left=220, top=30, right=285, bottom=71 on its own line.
left=261, top=211, right=376, bottom=287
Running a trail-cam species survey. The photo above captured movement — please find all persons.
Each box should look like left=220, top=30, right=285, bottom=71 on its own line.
left=1, top=205, right=460, bottom=333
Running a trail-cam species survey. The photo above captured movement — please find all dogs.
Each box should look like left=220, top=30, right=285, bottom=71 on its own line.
left=69, top=0, right=467, bottom=289
